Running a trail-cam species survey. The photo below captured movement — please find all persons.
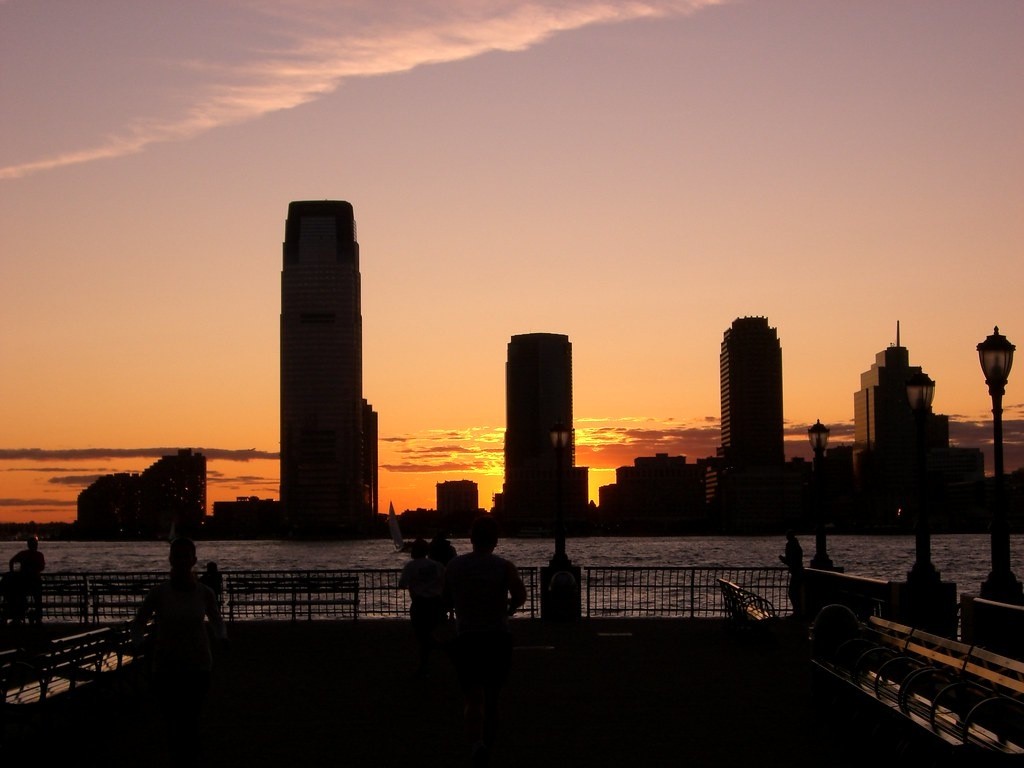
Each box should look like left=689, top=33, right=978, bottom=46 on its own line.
left=427, top=533, right=456, bottom=629
left=130, top=537, right=228, bottom=768
left=436, top=521, right=528, bottom=764
left=802, top=604, right=884, bottom=767
left=398, top=539, right=442, bottom=672
left=197, top=562, right=223, bottom=602
left=9, top=535, right=46, bottom=625
left=779, top=528, right=804, bottom=617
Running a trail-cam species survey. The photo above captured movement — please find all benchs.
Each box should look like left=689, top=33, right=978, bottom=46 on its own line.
left=717, top=579, right=779, bottom=628
left=0, top=616, right=150, bottom=729
left=806, top=615, right=1023, bottom=763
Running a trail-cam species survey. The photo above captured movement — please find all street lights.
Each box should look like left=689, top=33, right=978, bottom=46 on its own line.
left=806, top=416, right=834, bottom=572
left=976, top=327, right=1023, bottom=606
left=903, top=369, right=950, bottom=594
